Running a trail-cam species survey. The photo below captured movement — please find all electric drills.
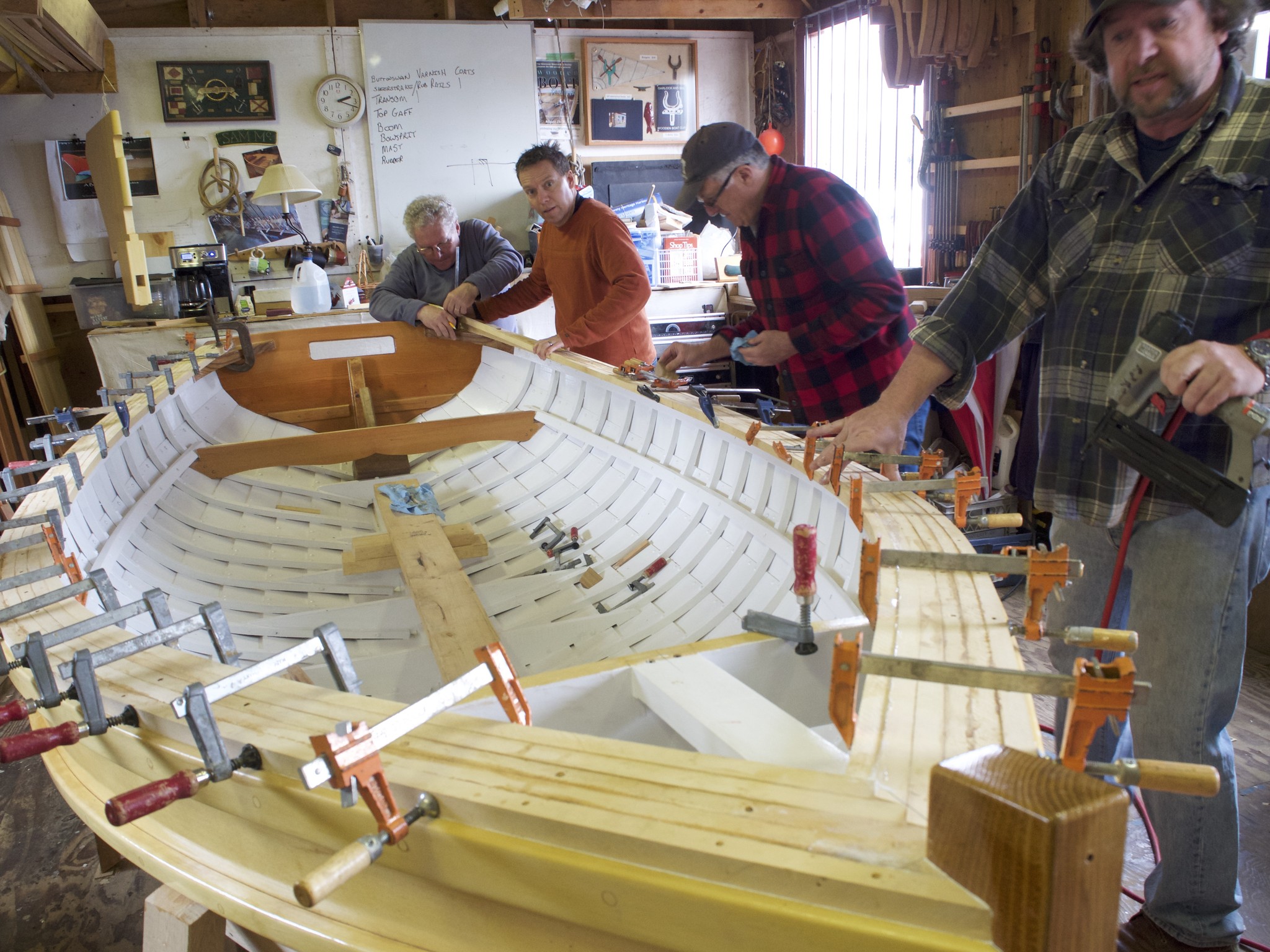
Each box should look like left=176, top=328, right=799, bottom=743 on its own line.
left=1080, top=309, right=1270, bottom=528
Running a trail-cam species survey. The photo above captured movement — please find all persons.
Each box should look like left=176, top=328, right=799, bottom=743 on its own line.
left=463, top=143, right=657, bottom=373
left=659, top=122, right=930, bottom=477
left=369, top=195, right=523, bottom=342
left=331, top=199, right=350, bottom=220
left=86, top=293, right=107, bottom=316
left=807, top=0, right=1270, bottom=952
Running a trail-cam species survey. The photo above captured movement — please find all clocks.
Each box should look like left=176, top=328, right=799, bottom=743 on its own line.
left=312, top=74, right=366, bottom=128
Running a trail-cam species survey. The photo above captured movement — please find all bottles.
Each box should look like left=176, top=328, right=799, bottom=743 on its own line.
left=982, top=414, right=1021, bottom=516
left=291, top=257, right=331, bottom=314
left=244, top=286, right=255, bottom=313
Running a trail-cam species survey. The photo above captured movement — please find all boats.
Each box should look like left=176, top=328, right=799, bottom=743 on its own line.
left=0, top=302, right=1130, bottom=952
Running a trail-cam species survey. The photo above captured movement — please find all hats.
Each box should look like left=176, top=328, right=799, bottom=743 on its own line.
left=1083, top=1, right=1121, bottom=43
left=673, top=122, right=755, bottom=212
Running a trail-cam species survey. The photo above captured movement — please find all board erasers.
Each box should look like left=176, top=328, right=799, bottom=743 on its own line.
left=367, top=239, right=376, bottom=247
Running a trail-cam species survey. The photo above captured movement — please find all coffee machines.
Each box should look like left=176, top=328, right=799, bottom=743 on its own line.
left=168, top=244, right=236, bottom=319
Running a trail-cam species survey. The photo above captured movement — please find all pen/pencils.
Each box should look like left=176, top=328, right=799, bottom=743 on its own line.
left=449, top=322, right=457, bottom=330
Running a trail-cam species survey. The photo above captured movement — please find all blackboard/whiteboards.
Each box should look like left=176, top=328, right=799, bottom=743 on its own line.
left=357, top=16, right=541, bottom=286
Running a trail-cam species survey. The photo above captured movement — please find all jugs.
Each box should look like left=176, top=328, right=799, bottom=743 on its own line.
left=172, top=274, right=214, bottom=308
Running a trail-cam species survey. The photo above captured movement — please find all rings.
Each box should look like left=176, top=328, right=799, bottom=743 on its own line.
left=455, top=308, right=459, bottom=311
left=548, top=341, right=553, bottom=346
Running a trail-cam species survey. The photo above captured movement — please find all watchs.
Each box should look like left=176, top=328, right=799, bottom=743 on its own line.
left=1243, top=339, right=1270, bottom=390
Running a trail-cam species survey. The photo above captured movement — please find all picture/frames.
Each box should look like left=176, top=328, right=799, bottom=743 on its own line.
left=580, top=37, right=700, bottom=146
left=156, top=59, right=276, bottom=124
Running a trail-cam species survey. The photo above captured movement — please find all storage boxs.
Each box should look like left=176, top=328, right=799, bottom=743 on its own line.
left=69, top=271, right=180, bottom=330
left=628, top=226, right=660, bottom=287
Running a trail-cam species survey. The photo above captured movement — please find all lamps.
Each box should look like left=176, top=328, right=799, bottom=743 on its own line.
left=250, top=162, right=323, bottom=248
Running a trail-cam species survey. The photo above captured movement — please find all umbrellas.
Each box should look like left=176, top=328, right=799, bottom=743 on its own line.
left=946, top=331, right=1023, bottom=510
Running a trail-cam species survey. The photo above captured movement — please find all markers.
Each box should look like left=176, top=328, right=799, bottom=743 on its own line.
left=380, top=234, right=383, bottom=245
left=366, top=236, right=376, bottom=250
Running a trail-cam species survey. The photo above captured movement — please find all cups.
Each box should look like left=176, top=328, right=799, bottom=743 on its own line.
left=285, top=246, right=305, bottom=270
left=325, top=244, right=346, bottom=264
left=312, top=247, right=326, bottom=269
left=248, top=249, right=270, bottom=275
left=366, top=242, right=384, bottom=265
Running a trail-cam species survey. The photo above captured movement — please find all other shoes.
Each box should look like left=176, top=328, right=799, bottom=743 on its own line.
left=1115, top=905, right=1241, bottom=952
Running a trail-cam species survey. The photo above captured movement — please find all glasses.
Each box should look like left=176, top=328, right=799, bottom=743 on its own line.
left=703, top=162, right=752, bottom=207
left=417, top=223, right=454, bottom=255
